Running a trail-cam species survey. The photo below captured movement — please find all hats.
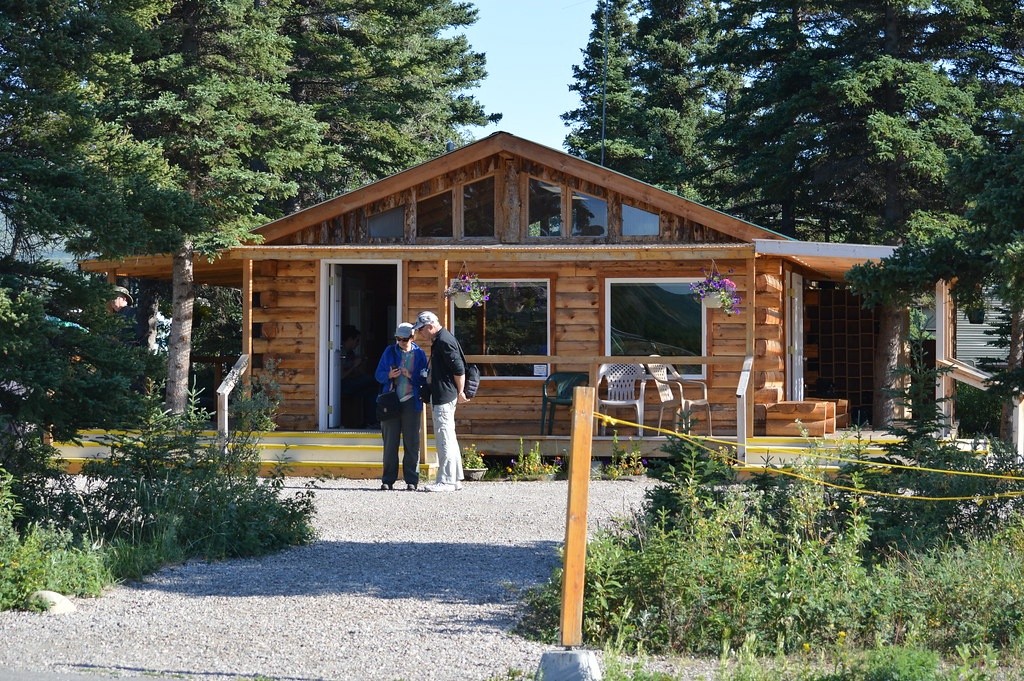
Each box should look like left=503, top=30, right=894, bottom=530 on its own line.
left=412, top=311, right=439, bottom=329
left=114, top=286, right=134, bottom=306
left=394, top=322, right=414, bottom=337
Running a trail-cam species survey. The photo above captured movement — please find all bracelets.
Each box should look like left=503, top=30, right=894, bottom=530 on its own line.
left=458, top=391, right=464, bottom=396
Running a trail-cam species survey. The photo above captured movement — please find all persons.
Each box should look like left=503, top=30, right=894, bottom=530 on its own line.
left=410, top=313, right=472, bottom=491
left=379, top=321, right=427, bottom=490
left=340, top=323, right=382, bottom=430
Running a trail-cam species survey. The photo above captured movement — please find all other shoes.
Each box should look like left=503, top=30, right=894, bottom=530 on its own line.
left=454, top=480, right=462, bottom=491
left=424, top=482, right=455, bottom=492
left=381, top=484, right=393, bottom=490
left=407, top=484, right=417, bottom=490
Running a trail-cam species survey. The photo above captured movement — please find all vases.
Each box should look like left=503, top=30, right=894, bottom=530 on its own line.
left=453, top=292, right=474, bottom=308
left=702, top=292, right=723, bottom=309
left=463, top=467, right=488, bottom=481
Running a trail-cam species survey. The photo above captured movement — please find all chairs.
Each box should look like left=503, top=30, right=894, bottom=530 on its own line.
left=646, top=354, right=713, bottom=437
left=598, top=363, right=647, bottom=437
left=540, top=371, right=590, bottom=435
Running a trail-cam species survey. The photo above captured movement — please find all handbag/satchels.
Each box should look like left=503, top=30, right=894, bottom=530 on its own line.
left=375, top=392, right=402, bottom=421
left=419, top=380, right=432, bottom=405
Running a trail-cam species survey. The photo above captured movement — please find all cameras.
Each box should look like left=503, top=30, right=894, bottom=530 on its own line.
left=420, top=385, right=432, bottom=403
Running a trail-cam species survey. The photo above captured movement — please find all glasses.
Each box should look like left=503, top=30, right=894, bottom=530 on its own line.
left=396, top=335, right=412, bottom=342
left=416, top=325, right=427, bottom=332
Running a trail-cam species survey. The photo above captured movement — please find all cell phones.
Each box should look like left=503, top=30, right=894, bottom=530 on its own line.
left=392, top=366, right=399, bottom=372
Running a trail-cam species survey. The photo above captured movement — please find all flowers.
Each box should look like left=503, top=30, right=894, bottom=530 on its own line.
left=599, top=429, right=648, bottom=482
left=505, top=436, right=565, bottom=483
left=687, top=267, right=744, bottom=316
left=442, top=273, right=490, bottom=308
left=460, top=443, right=486, bottom=469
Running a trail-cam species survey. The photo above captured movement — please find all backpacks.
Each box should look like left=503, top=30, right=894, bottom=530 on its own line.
left=455, top=338, right=480, bottom=399
